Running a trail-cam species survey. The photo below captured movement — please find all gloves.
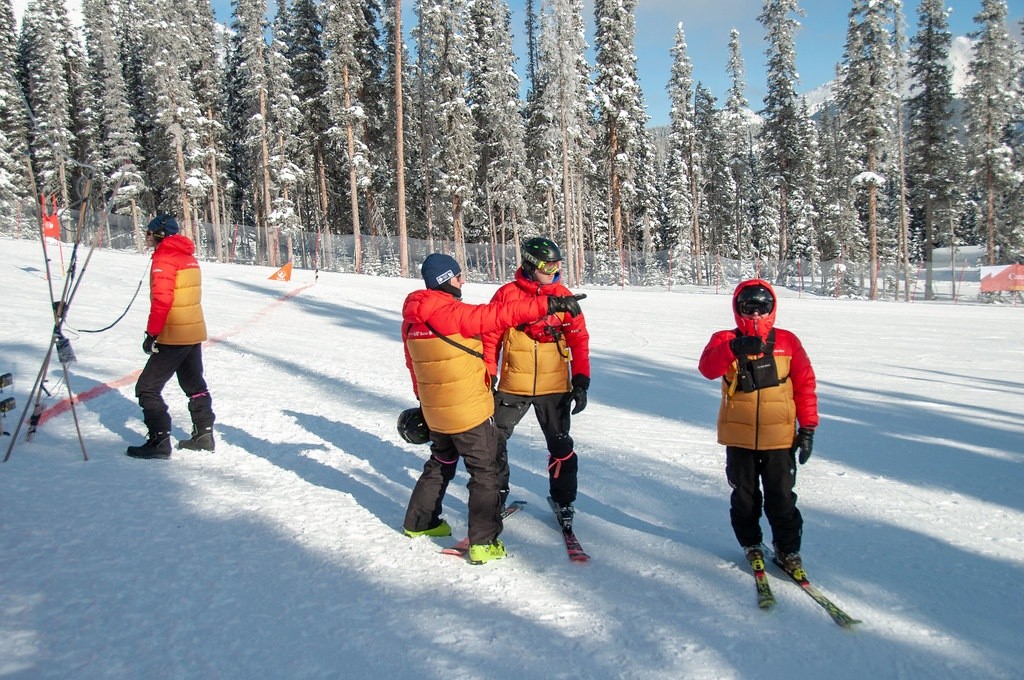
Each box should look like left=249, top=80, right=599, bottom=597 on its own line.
left=732, top=336, right=764, bottom=354
left=143, top=331, right=160, bottom=355
left=548, top=293, right=587, bottom=318
left=791, top=427, right=814, bottom=465
left=489, top=374, right=498, bottom=393
left=565, top=374, right=590, bottom=414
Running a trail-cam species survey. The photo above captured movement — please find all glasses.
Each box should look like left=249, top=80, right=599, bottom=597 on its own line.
left=147, top=231, right=154, bottom=236
left=541, top=260, right=562, bottom=276
left=741, top=302, right=766, bottom=314
left=455, top=274, right=461, bottom=282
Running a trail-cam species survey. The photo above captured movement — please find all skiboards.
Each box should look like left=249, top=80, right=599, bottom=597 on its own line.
left=440, top=495, right=592, bottom=560
left=752, top=542, right=863, bottom=626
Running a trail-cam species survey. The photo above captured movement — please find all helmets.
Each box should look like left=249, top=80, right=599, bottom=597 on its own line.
left=738, top=287, right=773, bottom=314
left=521, top=238, right=562, bottom=275
left=398, top=408, right=430, bottom=444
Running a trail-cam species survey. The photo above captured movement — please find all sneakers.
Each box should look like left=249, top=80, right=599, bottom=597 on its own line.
left=745, top=543, right=763, bottom=563
left=468, top=538, right=509, bottom=565
left=775, top=546, right=801, bottom=571
left=555, top=499, right=574, bottom=527
left=402, top=516, right=452, bottom=538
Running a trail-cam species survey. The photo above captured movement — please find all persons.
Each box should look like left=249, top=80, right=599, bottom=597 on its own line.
left=699, top=279, right=818, bottom=579
left=400, top=252, right=587, bottom=565
left=126, top=214, right=216, bottom=460
left=482, top=238, right=591, bottom=527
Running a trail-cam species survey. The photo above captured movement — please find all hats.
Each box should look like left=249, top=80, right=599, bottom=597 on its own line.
left=148, top=214, right=179, bottom=236
left=421, top=253, right=461, bottom=289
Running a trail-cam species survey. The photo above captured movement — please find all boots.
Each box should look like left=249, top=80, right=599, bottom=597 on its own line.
left=127, top=428, right=172, bottom=459
left=178, top=421, right=215, bottom=455
